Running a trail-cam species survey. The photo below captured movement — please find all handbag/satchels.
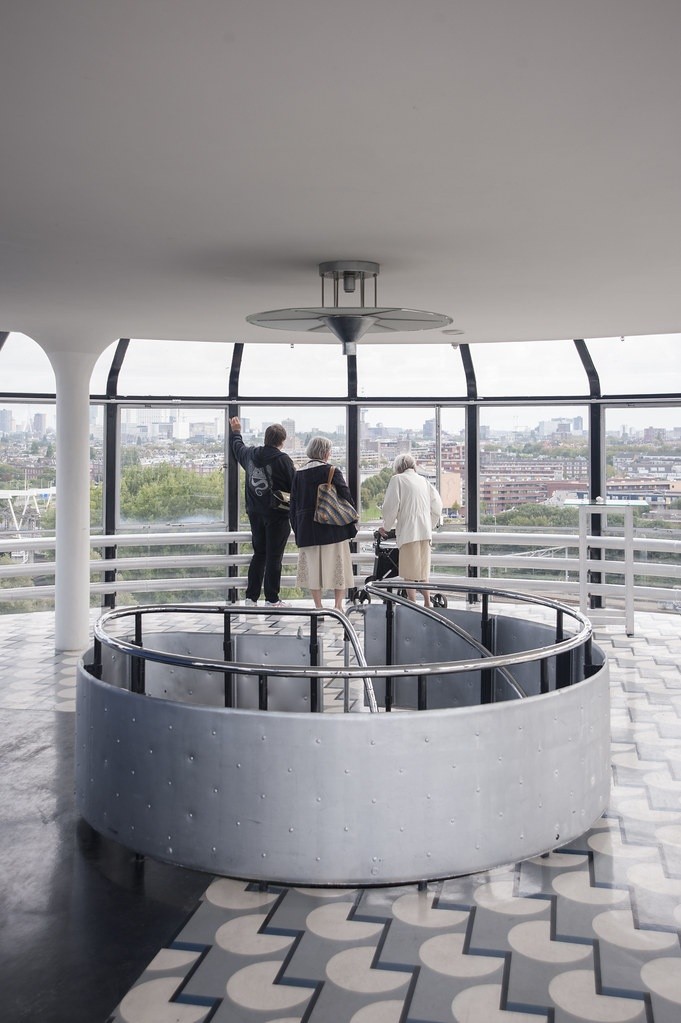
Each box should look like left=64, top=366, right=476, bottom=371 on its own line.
left=313, top=466, right=360, bottom=525
left=272, top=490, right=291, bottom=513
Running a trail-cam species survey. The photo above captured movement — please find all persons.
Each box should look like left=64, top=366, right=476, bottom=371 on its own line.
left=290, top=436, right=359, bottom=621
left=382, top=454, right=442, bottom=607
left=227, top=416, right=296, bottom=608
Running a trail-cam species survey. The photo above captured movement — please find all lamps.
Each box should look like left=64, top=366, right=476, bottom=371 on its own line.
left=244, top=260, right=453, bottom=355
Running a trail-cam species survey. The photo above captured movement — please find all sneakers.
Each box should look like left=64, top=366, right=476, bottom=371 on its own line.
left=245, top=598, right=258, bottom=607
left=265, top=600, right=292, bottom=608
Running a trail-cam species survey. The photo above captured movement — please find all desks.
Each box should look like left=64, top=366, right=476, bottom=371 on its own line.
left=563, top=498, right=649, bottom=637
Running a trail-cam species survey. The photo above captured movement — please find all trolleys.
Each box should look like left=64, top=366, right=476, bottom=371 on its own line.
left=352, top=528, right=448, bottom=608
